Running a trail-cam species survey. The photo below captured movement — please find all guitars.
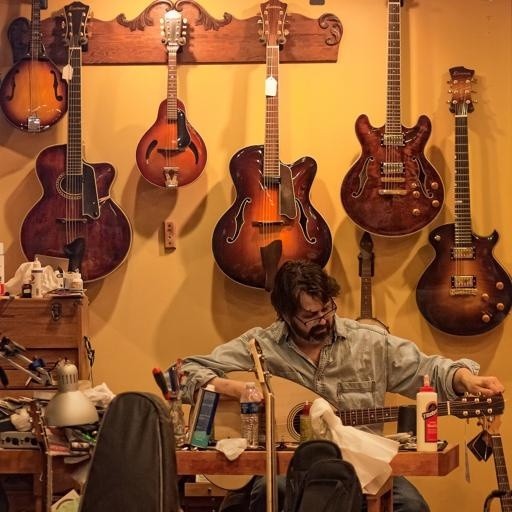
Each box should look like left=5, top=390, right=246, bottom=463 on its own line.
left=340, top=1, right=447, bottom=237
left=23, top=3, right=133, bottom=285
left=478, top=413, right=512, bottom=508
left=415, top=68, right=512, bottom=336
left=210, top=0, right=333, bottom=289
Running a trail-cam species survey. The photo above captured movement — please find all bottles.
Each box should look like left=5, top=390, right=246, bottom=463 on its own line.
left=22, top=280, right=32, bottom=296
left=239, top=381, right=261, bottom=450
left=416, top=374, right=438, bottom=453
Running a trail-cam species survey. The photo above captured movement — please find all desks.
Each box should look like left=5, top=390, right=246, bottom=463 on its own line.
left=176, top=442, right=463, bottom=512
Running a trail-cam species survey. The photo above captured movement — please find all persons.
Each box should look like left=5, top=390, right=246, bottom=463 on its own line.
left=164, top=258, right=505, bottom=512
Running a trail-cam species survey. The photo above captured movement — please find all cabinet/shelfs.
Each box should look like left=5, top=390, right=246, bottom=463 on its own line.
left=0, top=295, right=89, bottom=512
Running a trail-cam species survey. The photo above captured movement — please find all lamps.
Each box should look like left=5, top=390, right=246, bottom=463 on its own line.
left=0, top=334, right=98, bottom=428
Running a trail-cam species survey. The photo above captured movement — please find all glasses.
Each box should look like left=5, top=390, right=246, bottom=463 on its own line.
left=288, top=296, right=338, bottom=328
